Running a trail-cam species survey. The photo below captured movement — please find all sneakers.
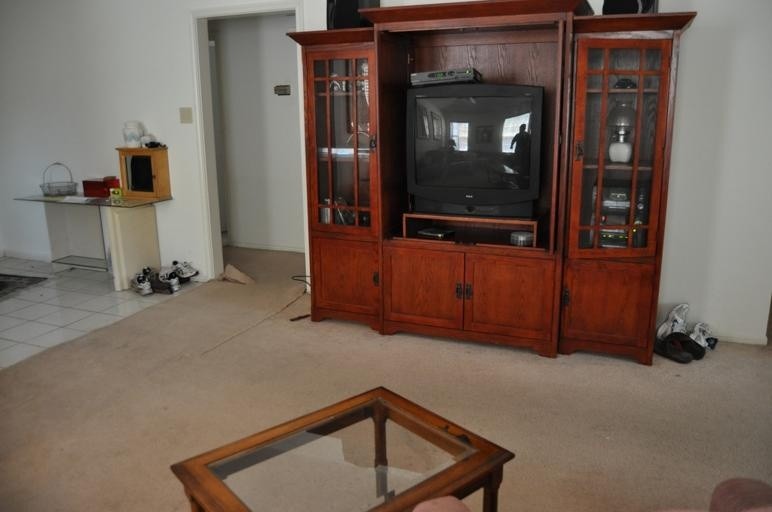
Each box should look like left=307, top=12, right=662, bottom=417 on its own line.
left=656, top=303, right=690, bottom=342
left=689, top=322, right=713, bottom=349
left=130, top=260, right=198, bottom=297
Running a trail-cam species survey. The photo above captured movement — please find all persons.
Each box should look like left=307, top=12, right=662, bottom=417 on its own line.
left=510, top=123, right=531, bottom=170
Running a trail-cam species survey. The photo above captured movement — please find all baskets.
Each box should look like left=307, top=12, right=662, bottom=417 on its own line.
left=40, top=163, right=76, bottom=197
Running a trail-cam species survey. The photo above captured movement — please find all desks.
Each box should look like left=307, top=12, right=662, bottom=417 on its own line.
left=10, top=187, right=169, bottom=291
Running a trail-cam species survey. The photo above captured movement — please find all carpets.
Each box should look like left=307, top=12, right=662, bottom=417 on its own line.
left=0, top=273, right=50, bottom=302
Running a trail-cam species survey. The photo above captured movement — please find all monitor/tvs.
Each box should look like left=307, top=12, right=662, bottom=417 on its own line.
left=405, top=83, right=544, bottom=220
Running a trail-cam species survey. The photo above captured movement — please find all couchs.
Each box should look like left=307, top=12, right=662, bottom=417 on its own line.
left=410, top=475, right=768, bottom=511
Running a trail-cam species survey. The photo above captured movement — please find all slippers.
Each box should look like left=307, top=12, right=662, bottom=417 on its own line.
left=653, top=338, right=694, bottom=363
left=664, top=332, right=705, bottom=361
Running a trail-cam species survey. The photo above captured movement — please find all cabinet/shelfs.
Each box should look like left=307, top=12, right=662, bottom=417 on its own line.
left=284, top=26, right=379, bottom=327
left=117, top=146, right=171, bottom=200
left=558, top=11, right=701, bottom=368
left=361, top=0, right=587, bottom=360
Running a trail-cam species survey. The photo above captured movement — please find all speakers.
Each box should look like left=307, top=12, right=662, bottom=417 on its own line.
left=326, top=0, right=379, bottom=29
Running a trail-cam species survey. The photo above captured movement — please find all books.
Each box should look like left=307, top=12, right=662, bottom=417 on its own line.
left=319, top=196, right=357, bottom=226
left=416, top=227, right=455, bottom=238
